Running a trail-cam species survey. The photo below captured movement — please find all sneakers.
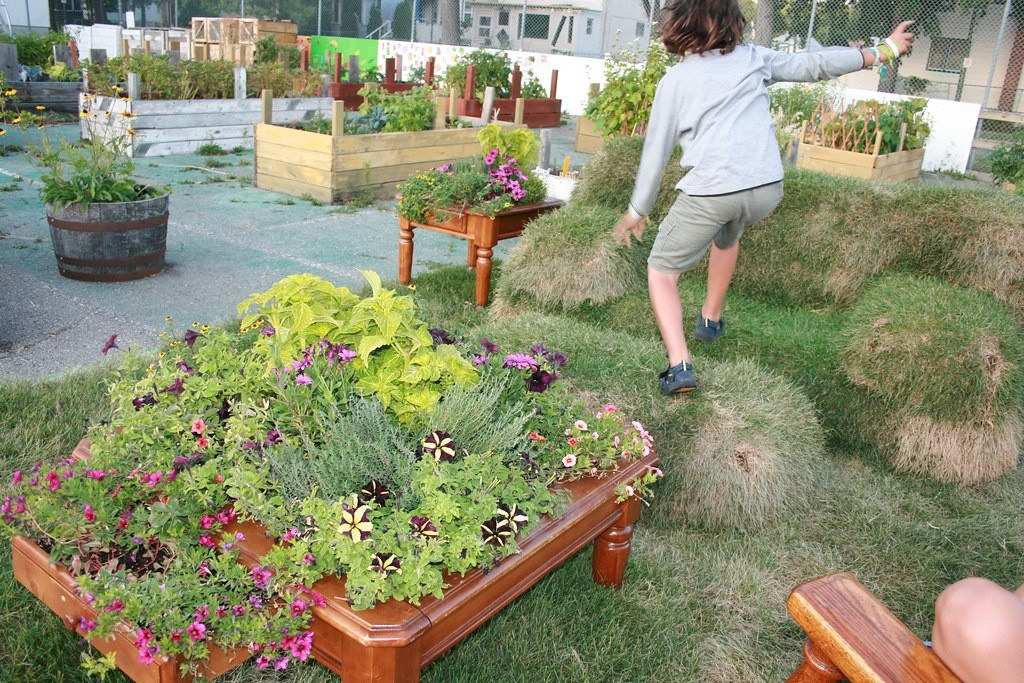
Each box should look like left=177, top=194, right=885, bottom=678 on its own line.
left=695, top=314, right=726, bottom=342
left=657, top=360, right=698, bottom=395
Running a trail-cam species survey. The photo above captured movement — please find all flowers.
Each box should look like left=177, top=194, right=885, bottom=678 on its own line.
left=0, top=82, right=173, bottom=216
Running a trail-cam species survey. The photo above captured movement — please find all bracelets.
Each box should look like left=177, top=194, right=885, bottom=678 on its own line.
left=628, top=201, right=651, bottom=227
left=865, top=37, right=900, bottom=76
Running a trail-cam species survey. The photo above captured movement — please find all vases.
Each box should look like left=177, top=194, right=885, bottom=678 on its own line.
left=46, top=180, right=172, bottom=283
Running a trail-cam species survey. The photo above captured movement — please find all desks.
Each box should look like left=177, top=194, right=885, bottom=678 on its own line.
left=13, top=412, right=662, bottom=683
left=395, top=190, right=567, bottom=313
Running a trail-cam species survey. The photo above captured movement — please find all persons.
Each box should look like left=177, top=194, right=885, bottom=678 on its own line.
left=932, top=579, right=1024, bottom=683
left=616, top=1, right=916, bottom=395
